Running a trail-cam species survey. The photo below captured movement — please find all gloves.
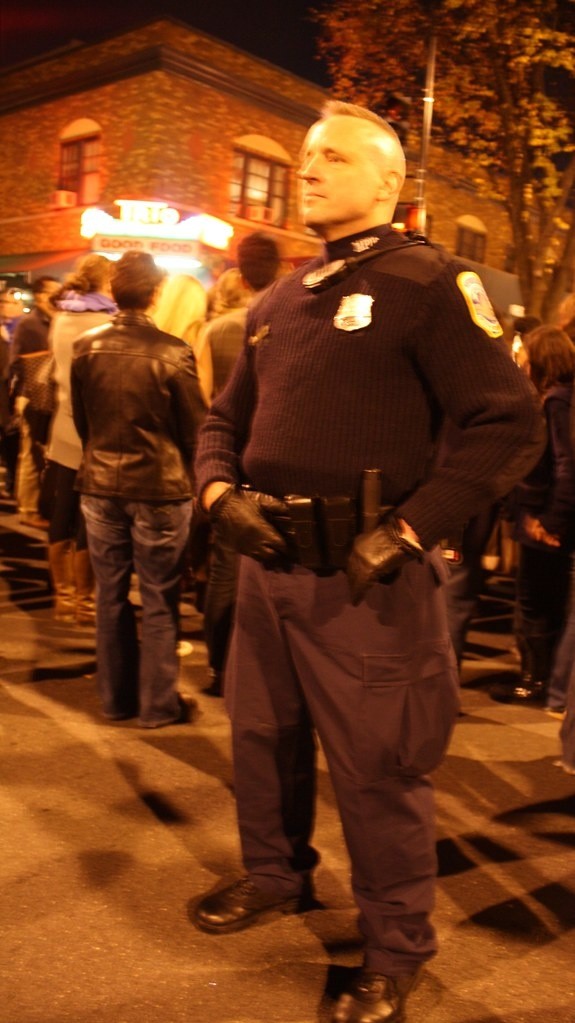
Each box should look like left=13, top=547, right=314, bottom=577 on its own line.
left=211, top=484, right=292, bottom=564
left=344, top=517, right=425, bottom=607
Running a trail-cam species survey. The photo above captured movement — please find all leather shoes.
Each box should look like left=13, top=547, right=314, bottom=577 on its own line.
left=333, top=960, right=427, bottom=1023
left=197, top=877, right=317, bottom=933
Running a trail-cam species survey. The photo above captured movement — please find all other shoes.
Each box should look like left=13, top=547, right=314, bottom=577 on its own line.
left=20, top=512, right=51, bottom=530
left=56, top=599, right=99, bottom=626
left=101, top=705, right=141, bottom=719
left=140, top=695, right=198, bottom=728
left=491, top=676, right=549, bottom=707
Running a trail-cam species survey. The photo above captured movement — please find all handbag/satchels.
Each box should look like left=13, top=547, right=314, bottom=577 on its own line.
left=21, top=349, right=58, bottom=412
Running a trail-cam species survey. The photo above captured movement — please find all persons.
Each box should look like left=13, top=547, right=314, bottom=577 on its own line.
left=0, top=233, right=280, bottom=696
left=194, top=104, right=546, bottom=1023
left=69, top=251, right=209, bottom=728
left=46, top=255, right=118, bottom=625
left=441, top=315, right=575, bottom=774
